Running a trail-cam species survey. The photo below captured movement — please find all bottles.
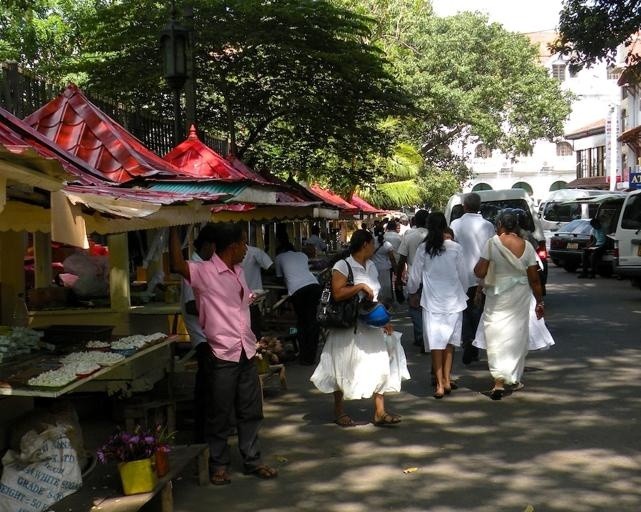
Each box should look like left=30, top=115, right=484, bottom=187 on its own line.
left=11, top=293, right=28, bottom=329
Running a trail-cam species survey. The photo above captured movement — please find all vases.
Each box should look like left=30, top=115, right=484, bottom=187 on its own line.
left=256, top=355, right=270, bottom=374
left=154, top=452, right=169, bottom=478
left=115, top=454, right=160, bottom=497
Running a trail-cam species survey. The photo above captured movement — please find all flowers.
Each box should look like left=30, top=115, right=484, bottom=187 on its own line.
left=156, top=425, right=178, bottom=455
left=253, top=337, right=283, bottom=363
left=95, top=424, right=155, bottom=464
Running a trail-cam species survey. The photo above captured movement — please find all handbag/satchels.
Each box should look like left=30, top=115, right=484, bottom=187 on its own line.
left=315, top=258, right=359, bottom=335
left=479, top=239, right=496, bottom=288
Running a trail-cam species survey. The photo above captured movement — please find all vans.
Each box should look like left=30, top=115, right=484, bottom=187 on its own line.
left=611, top=188, right=641, bottom=287
left=444, top=186, right=548, bottom=293
left=534, top=186, right=617, bottom=230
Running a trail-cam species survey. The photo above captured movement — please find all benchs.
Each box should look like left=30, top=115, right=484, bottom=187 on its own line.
left=259, top=363, right=289, bottom=404
left=42, top=443, right=211, bottom=512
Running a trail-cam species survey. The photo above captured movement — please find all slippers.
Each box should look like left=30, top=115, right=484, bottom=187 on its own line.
left=432, top=375, right=458, bottom=398
left=209, top=470, right=231, bottom=485
left=511, top=382, right=525, bottom=391
left=253, top=467, right=278, bottom=479
left=490, top=386, right=504, bottom=400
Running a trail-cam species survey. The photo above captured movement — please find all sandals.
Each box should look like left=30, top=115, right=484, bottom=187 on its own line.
left=374, top=413, right=401, bottom=426
left=335, top=413, right=355, bottom=425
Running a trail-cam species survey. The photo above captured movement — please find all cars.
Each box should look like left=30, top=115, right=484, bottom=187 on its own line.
left=551, top=216, right=604, bottom=272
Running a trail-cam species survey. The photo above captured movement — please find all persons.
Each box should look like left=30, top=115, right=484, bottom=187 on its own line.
left=576, top=218, right=614, bottom=280
left=165, top=221, right=281, bottom=487
left=237, top=191, right=557, bottom=428
left=177, top=222, right=256, bottom=445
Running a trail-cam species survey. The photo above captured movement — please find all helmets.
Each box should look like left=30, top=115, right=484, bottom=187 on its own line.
left=358, top=296, right=394, bottom=326
